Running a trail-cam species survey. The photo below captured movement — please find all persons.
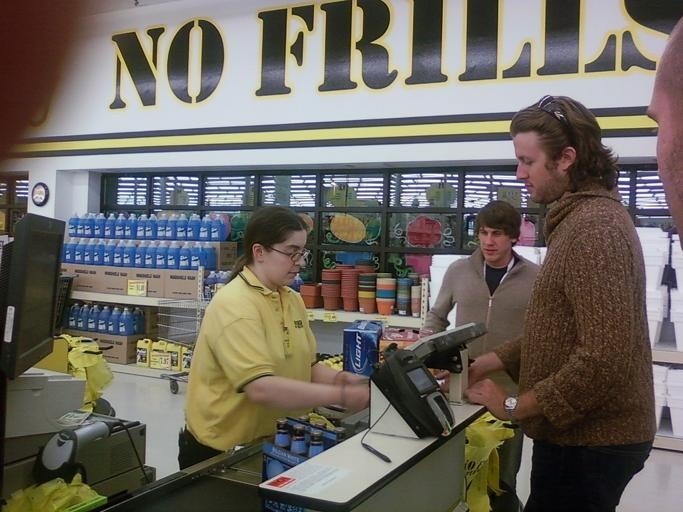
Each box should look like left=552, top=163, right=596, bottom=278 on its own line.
left=2, top=0, right=111, bottom=171
left=177, top=203, right=369, bottom=473
left=418, top=197, right=543, bottom=512
left=461, top=91, right=657, bottom=512
left=643, top=14, right=682, bottom=254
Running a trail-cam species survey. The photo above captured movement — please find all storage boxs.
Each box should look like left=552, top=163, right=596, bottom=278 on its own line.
left=63, top=237, right=238, bottom=365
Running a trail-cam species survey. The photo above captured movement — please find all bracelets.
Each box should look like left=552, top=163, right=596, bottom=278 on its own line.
left=333, top=371, right=343, bottom=385
left=339, top=383, right=345, bottom=411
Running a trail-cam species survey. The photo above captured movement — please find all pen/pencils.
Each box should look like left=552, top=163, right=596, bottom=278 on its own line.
left=362, top=442, right=391, bottom=463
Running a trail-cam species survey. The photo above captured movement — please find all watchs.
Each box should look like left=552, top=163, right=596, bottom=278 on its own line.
left=501, top=395, right=520, bottom=423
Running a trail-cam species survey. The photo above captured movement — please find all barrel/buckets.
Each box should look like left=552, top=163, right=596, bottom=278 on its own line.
left=67, top=303, right=146, bottom=336
left=135, top=338, right=192, bottom=371
left=62, top=209, right=232, bottom=300
left=291, top=273, right=304, bottom=290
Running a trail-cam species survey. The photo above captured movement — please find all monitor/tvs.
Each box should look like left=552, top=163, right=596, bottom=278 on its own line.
left=404, top=320, right=489, bottom=363
left=1, top=212, right=66, bottom=382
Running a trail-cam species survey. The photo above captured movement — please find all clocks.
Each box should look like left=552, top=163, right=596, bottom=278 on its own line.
left=32, top=182, right=50, bottom=207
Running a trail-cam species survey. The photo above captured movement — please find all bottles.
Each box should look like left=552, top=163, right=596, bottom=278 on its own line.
left=65, top=303, right=145, bottom=336
left=275, top=416, right=346, bottom=459
left=62, top=212, right=227, bottom=270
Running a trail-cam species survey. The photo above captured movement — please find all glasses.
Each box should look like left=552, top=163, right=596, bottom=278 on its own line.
left=263, top=246, right=310, bottom=261
left=538, top=93, right=574, bottom=139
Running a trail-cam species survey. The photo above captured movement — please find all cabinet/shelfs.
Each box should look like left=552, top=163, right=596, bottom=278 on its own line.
left=69, top=291, right=683, bottom=452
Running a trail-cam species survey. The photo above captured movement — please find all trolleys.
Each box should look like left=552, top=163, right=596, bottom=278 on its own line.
left=158, top=297, right=213, bottom=394
